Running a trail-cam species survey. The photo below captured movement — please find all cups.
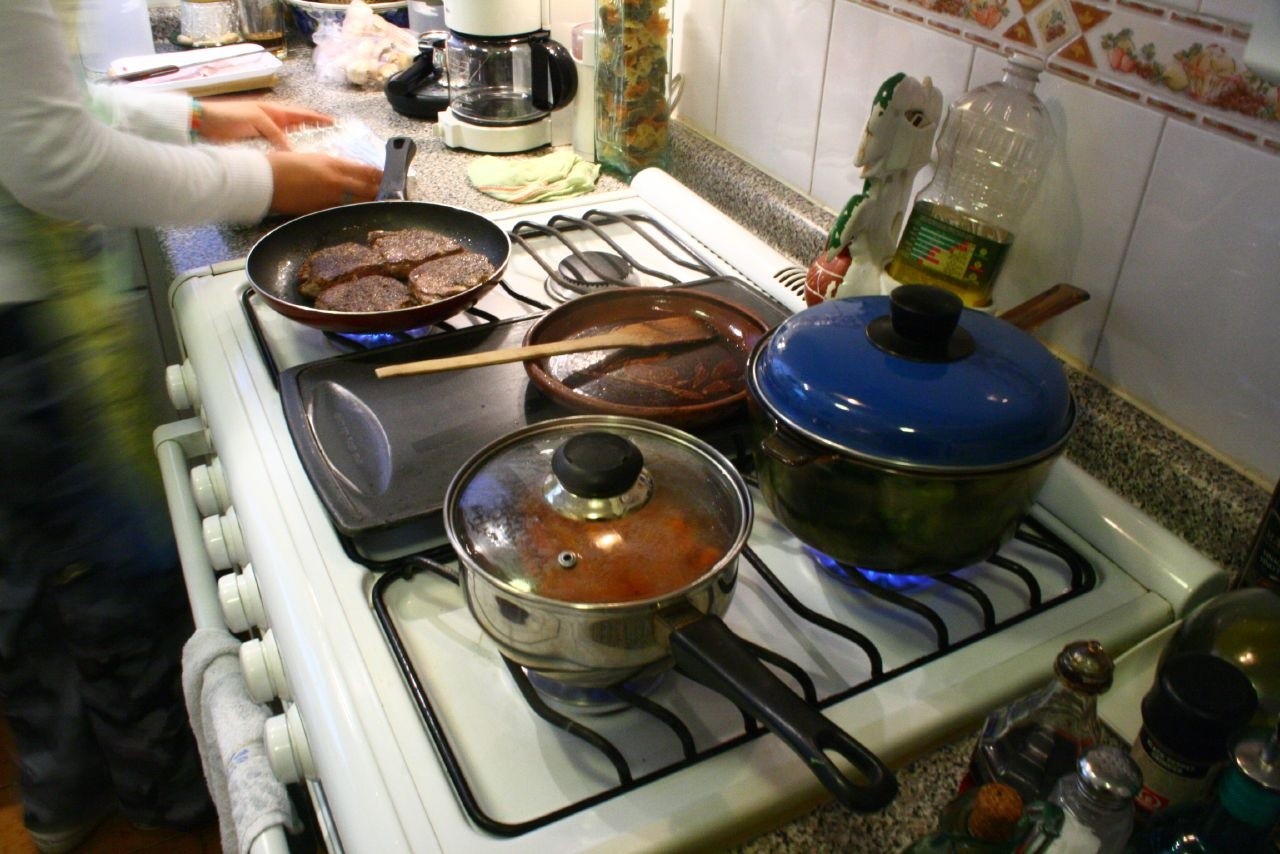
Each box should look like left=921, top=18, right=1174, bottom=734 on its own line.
left=237, top=0, right=286, bottom=52
left=73, top=0, right=156, bottom=82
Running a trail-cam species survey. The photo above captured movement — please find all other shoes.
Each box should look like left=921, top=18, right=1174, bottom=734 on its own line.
left=24, top=790, right=113, bottom=854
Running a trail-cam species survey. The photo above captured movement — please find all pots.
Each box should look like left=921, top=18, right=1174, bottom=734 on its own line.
left=243, top=134, right=511, bottom=337
left=745, top=278, right=1091, bottom=575
left=443, top=413, right=899, bottom=812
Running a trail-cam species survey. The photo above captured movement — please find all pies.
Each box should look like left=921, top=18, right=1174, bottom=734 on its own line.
left=297, top=228, right=497, bottom=311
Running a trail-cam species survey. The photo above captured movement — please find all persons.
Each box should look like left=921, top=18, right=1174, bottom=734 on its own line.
left=0, top=0, right=384, bottom=854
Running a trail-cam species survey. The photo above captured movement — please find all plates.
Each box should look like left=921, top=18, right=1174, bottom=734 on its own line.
left=107, top=42, right=284, bottom=100
left=519, top=284, right=770, bottom=419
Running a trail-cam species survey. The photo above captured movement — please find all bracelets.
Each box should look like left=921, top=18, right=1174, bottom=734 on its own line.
left=189, top=98, right=204, bottom=141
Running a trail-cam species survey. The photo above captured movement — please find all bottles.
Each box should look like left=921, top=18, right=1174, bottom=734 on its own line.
left=901, top=565, right=1280, bottom=854
left=891, top=50, right=1058, bottom=302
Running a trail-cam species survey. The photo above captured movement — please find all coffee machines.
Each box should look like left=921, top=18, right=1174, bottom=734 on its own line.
left=434, top=0, right=578, bottom=155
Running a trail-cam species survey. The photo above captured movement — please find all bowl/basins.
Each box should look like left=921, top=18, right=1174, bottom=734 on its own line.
left=404, top=0, right=442, bottom=33
left=284, top=0, right=407, bottom=40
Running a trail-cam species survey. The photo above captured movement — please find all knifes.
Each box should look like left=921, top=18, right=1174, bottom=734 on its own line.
left=94, top=45, right=284, bottom=82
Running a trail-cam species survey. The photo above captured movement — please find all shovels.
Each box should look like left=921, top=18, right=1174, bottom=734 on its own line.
left=374, top=313, right=721, bottom=380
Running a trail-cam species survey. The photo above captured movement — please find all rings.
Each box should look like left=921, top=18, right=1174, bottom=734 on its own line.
left=343, top=176, right=354, bottom=201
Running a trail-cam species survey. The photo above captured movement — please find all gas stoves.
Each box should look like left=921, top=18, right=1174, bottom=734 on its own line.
left=164, top=166, right=1232, bottom=854
